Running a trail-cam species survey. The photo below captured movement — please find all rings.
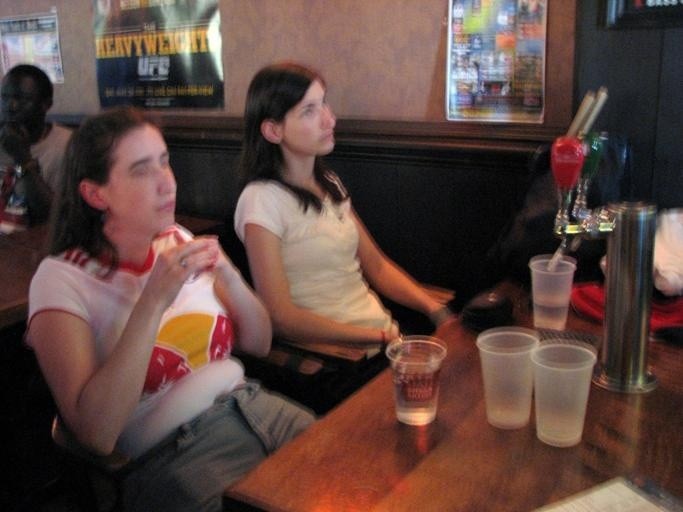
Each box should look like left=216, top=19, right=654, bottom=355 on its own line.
left=179, top=258, right=187, bottom=268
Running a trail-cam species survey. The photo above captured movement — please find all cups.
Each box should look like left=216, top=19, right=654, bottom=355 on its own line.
left=473, top=326, right=598, bottom=449
left=387, top=335, right=447, bottom=430
left=526, top=253, right=576, bottom=330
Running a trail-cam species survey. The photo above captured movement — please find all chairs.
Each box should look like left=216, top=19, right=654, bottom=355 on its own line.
left=246, top=284, right=455, bottom=398
left=44, top=410, right=140, bottom=509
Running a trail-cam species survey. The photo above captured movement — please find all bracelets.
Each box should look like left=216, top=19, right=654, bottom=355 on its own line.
left=378, top=330, right=386, bottom=348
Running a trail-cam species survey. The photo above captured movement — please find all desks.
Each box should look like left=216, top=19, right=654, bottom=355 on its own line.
left=222, top=254, right=682, bottom=508
left=0, top=206, right=223, bottom=325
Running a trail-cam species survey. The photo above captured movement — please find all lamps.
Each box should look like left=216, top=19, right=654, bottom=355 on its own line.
left=552, top=132, right=604, bottom=226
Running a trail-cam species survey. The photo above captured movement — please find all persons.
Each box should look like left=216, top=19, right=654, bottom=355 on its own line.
left=0, top=64, right=71, bottom=233
left=232, top=64, right=454, bottom=373
left=24, top=106, right=317, bottom=512
